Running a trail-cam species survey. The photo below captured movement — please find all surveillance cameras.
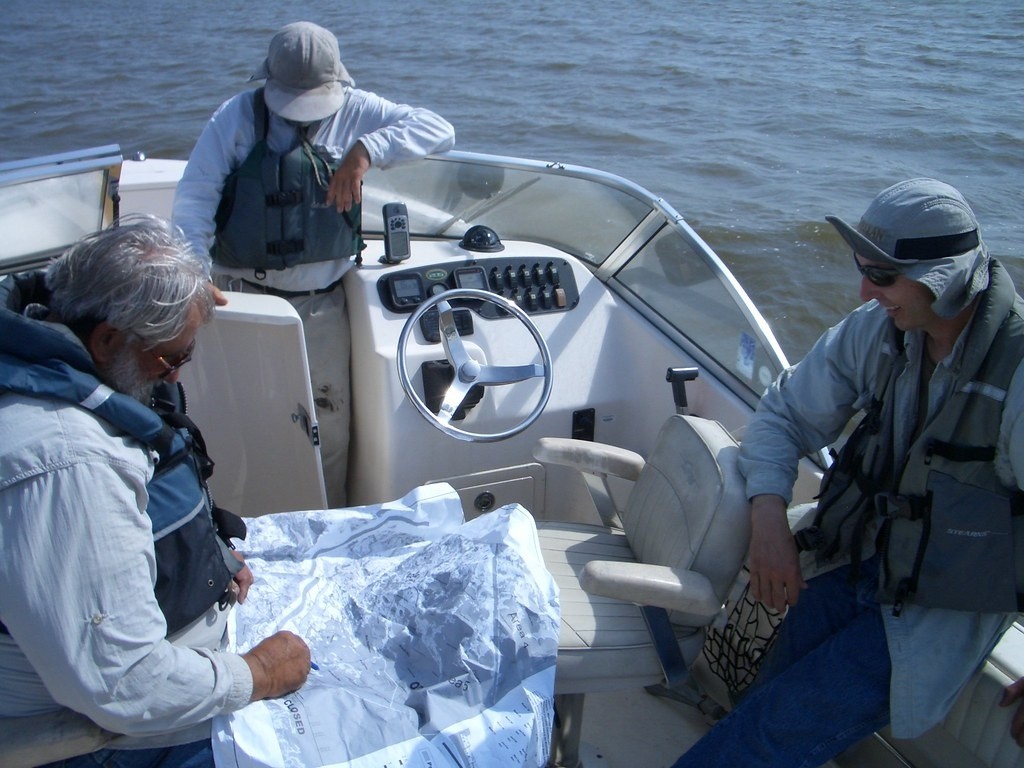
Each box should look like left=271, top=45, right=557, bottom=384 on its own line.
left=459, top=224, right=505, bottom=252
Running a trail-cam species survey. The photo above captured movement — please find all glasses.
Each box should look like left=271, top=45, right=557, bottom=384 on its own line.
left=129, top=327, right=195, bottom=380
left=853, top=252, right=903, bottom=287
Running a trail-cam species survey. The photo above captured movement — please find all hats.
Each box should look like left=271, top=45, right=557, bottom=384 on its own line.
left=825, top=178, right=991, bottom=321
left=247, top=20, right=356, bottom=122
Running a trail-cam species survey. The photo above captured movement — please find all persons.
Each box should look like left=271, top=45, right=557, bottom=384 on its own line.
left=668, top=175, right=1024, bottom=767
left=168, top=19, right=455, bottom=509
left=1, top=211, right=312, bottom=768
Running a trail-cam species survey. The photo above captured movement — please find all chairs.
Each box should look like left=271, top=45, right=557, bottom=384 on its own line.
left=533, top=414, right=752, bottom=693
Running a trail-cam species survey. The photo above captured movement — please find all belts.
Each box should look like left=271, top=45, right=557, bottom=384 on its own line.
left=241, top=279, right=341, bottom=299
